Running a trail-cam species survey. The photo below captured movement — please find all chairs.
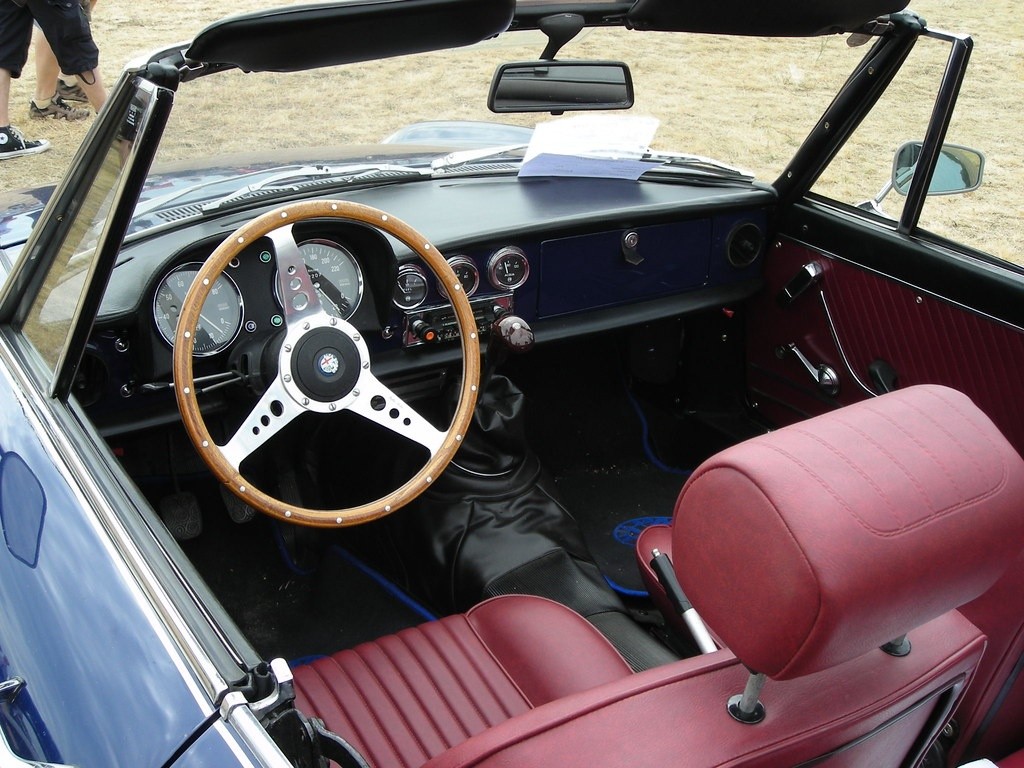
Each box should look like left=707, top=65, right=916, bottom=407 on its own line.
left=289, top=377, right=1023, bottom=767
left=639, top=524, right=1024, bottom=768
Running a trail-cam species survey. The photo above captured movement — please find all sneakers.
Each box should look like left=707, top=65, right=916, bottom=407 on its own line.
left=54, top=76, right=89, bottom=102
left=28, top=90, right=90, bottom=122
left=0, top=124, right=51, bottom=160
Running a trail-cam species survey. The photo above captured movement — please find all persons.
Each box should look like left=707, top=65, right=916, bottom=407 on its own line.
left=30, top=1, right=98, bottom=120
left=0, top=0, right=108, bottom=160
